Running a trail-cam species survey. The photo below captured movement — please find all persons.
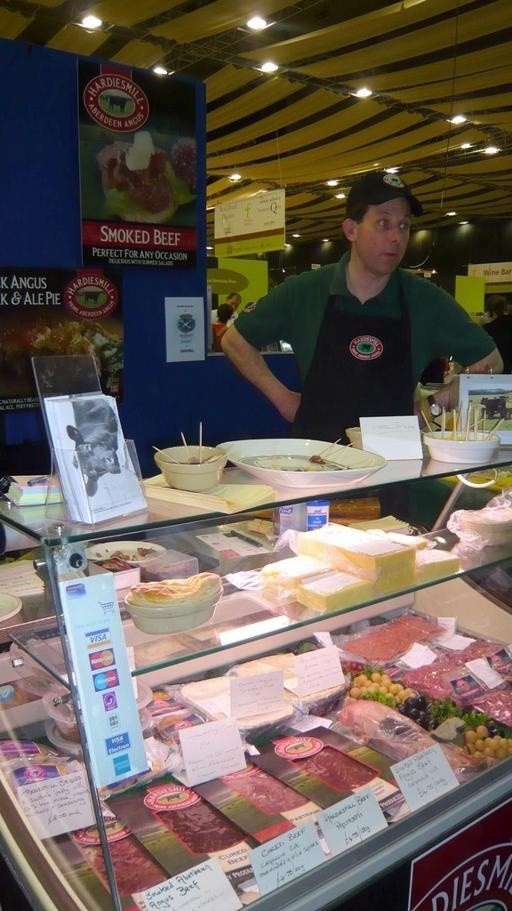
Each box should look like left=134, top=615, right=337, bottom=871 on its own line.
left=211, top=293, right=242, bottom=352
left=481, top=294, right=512, bottom=375
left=219, top=170, right=504, bottom=524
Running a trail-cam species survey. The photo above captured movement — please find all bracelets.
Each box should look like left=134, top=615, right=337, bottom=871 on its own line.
left=124, top=584, right=224, bottom=635
left=152, top=445, right=228, bottom=493
left=344, top=426, right=363, bottom=451
left=423, top=431, right=501, bottom=464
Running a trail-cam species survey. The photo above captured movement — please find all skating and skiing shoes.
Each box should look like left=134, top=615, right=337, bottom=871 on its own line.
left=350, top=673, right=512, bottom=761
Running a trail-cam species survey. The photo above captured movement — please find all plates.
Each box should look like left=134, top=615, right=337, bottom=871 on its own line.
left=0, top=428, right=512, bottom=911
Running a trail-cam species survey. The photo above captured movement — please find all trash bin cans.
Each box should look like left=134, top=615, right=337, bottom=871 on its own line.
left=426, top=395, right=441, bottom=418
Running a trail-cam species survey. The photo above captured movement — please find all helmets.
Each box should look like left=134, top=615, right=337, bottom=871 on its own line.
left=83, top=540, right=167, bottom=568
left=215, top=438, right=388, bottom=489
left=101, top=158, right=182, bottom=223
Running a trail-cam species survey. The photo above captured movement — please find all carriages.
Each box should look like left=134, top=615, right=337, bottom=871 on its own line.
left=261, top=522, right=460, bottom=614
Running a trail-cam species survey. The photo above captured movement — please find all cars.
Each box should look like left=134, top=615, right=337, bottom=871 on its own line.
left=272, top=503, right=308, bottom=541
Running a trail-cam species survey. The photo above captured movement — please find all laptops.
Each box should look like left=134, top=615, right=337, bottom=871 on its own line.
left=127, top=571, right=222, bottom=607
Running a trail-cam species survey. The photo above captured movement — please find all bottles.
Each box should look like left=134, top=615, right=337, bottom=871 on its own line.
left=348, top=172, right=424, bottom=217
